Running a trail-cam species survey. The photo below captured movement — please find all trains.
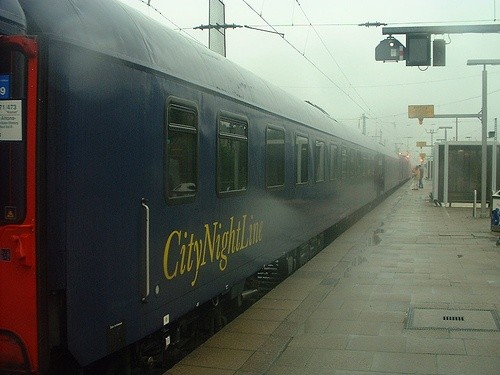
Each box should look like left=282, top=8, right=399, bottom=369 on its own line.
left=0, top=1, right=413, bottom=374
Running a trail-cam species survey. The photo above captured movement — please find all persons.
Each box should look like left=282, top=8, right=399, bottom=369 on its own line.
left=418, top=164, right=424, bottom=188
left=411, top=166, right=420, bottom=190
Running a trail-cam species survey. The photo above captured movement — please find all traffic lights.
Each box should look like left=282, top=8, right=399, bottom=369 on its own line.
left=406, top=33, right=431, bottom=67
